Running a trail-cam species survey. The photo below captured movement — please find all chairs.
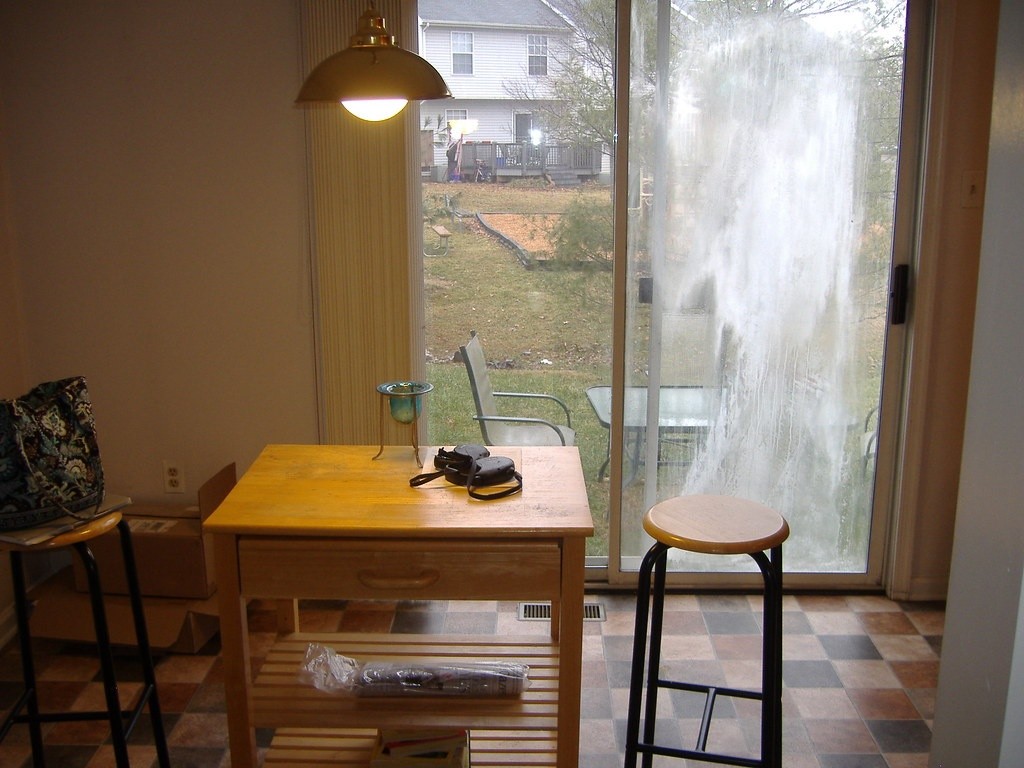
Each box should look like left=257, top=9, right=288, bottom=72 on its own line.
left=459, top=329, right=577, bottom=446
left=854, top=405, right=879, bottom=523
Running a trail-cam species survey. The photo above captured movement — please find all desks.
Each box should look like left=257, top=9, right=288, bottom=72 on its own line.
left=585, top=385, right=733, bottom=492
left=201, top=444, right=594, bottom=768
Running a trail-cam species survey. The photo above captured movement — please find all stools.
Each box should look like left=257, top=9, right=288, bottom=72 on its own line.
left=624, top=493, right=790, bottom=768
left=0, top=511, right=171, bottom=768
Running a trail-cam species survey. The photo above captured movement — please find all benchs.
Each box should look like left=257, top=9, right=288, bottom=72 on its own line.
left=431, top=225, right=452, bottom=256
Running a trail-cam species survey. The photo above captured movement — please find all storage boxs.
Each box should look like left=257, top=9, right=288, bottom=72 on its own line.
left=72, top=462, right=236, bottom=599
left=28, top=565, right=253, bottom=654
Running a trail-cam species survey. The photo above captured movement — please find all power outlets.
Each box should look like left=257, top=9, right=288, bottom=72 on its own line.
left=165, top=463, right=185, bottom=494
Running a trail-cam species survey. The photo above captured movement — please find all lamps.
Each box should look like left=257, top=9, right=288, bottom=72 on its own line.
left=293, top=0, right=455, bottom=122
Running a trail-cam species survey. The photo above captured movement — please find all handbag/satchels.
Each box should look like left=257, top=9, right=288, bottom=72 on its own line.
left=0, top=376, right=105, bottom=531
